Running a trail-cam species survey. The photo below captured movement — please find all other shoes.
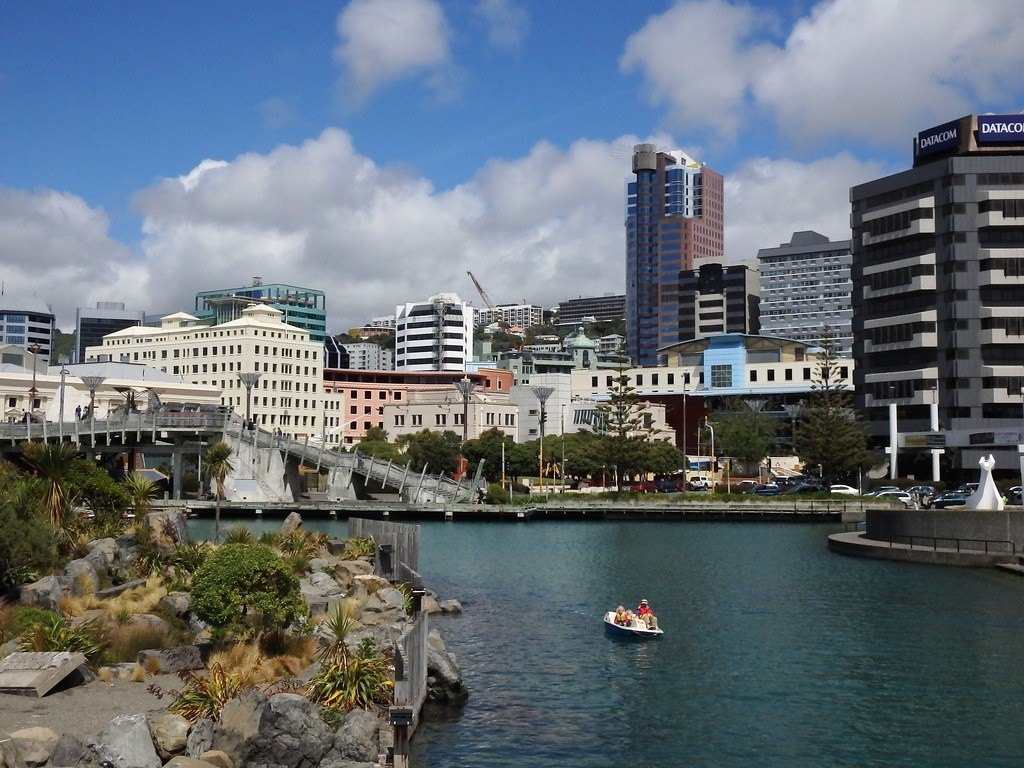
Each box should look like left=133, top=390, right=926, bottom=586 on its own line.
left=649, top=626, right=655, bottom=630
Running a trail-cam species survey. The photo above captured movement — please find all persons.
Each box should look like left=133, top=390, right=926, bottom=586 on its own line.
left=969, top=488, right=975, bottom=496
left=911, top=490, right=920, bottom=510
left=634, top=598, right=656, bottom=630
left=614, top=605, right=631, bottom=627
left=1000, top=493, right=1006, bottom=510
left=76, top=405, right=81, bottom=420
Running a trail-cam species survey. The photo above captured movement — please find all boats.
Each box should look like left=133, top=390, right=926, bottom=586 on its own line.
left=605, top=609, right=662, bottom=638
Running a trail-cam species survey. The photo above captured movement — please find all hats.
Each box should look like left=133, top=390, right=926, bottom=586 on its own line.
left=641, top=599, right=648, bottom=604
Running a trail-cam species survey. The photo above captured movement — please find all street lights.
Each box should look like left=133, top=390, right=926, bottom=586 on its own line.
left=82, top=377, right=106, bottom=421
left=532, top=387, right=555, bottom=493
left=452, top=373, right=478, bottom=479
left=239, top=372, right=259, bottom=431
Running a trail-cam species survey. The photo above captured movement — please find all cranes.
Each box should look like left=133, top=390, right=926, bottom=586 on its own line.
left=467, top=271, right=520, bottom=350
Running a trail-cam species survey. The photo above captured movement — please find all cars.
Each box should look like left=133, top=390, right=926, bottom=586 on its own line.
left=631, top=482, right=658, bottom=493
left=689, top=477, right=718, bottom=492
left=862, top=485, right=1023, bottom=509
left=738, top=475, right=859, bottom=496
left=655, top=476, right=694, bottom=492
